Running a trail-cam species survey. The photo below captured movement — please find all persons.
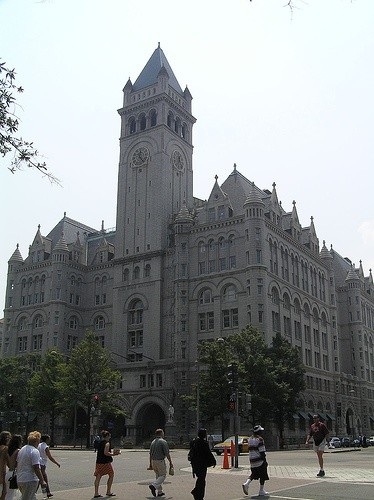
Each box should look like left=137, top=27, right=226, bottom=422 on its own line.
left=94, top=430, right=116, bottom=498
left=169, top=405, right=174, bottom=420
left=305, top=415, right=329, bottom=476
left=190, top=429, right=216, bottom=500
left=16, top=430, right=47, bottom=500
left=358, top=435, right=368, bottom=448
left=242, top=425, right=270, bottom=496
left=0, top=431, right=23, bottom=500
left=149, top=429, right=174, bottom=497
left=37, top=435, right=60, bottom=498
left=208, top=434, right=213, bottom=440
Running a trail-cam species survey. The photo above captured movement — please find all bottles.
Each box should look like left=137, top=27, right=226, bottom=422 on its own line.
left=42, top=487, right=47, bottom=499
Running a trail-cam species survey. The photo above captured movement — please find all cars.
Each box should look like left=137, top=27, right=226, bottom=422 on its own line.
left=341, top=437, right=374, bottom=448
left=326, top=437, right=342, bottom=448
left=212, top=436, right=250, bottom=456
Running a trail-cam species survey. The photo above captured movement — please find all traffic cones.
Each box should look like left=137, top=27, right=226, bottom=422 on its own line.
left=229, top=440, right=236, bottom=456
left=220, top=446, right=231, bottom=469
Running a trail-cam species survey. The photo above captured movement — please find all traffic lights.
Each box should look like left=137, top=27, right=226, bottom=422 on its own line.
left=237, top=391, right=243, bottom=399
left=228, top=364, right=235, bottom=386
left=228, top=394, right=236, bottom=411
left=94, top=394, right=100, bottom=407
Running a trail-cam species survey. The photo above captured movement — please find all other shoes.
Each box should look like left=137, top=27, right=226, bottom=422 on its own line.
left=158, top=492, right=165, bottom=496
left=259, top=490, right=270, bottom=496
left=317, top=470, right=325, bottom=476
left=149, top=485, right=157, bottom=497
left=190, top=489, right=204, bottom=500
left=242, top=483, right=249, bottom=495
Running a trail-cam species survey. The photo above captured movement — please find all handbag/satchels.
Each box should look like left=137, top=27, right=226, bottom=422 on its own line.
left=7, top=469, right=18, bottom=489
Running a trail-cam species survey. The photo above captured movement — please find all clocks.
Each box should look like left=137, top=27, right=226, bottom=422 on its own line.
left=131, top=146, right=150, bottom=169
left=171, top=150, right=184, bottom=171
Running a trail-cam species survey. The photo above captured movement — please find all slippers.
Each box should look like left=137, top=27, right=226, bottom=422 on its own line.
left=106, top=493, right=116, bottom=496
left=95, top=493, right=103, bottom=497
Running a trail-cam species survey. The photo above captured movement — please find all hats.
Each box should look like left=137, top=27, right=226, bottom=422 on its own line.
left=253, top=425, right=264, bottom=432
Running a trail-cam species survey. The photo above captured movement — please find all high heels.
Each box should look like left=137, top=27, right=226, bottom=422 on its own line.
left=47, top=493, right=53, bottom=499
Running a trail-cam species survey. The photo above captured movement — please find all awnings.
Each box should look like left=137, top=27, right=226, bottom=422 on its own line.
left=370, top=416, right=374, bottom=422
left=291, top=411, right=308, bottom=420
left=308, top=412, right=336, bottom=421
